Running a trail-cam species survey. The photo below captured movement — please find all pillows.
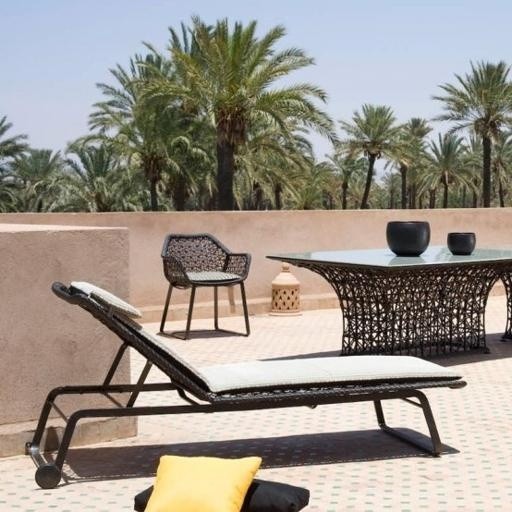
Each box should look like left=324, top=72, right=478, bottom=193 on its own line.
left=144, top=456, right=262, bottom=512
left=134, top=479, right=309, bottom=512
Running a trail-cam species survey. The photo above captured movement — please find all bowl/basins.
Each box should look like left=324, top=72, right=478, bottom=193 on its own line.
left=447, top=232, right=476, bottom=255
left=386, top=221, right=430, bottom=257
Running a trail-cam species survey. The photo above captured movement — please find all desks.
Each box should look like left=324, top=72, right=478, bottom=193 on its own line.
left=266, top=245, right=512, bottom=356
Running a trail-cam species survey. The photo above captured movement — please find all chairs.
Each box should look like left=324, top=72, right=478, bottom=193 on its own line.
left=25, top=281, right=467, bottom=489
left=160, top=233, right=251, bottom=340
